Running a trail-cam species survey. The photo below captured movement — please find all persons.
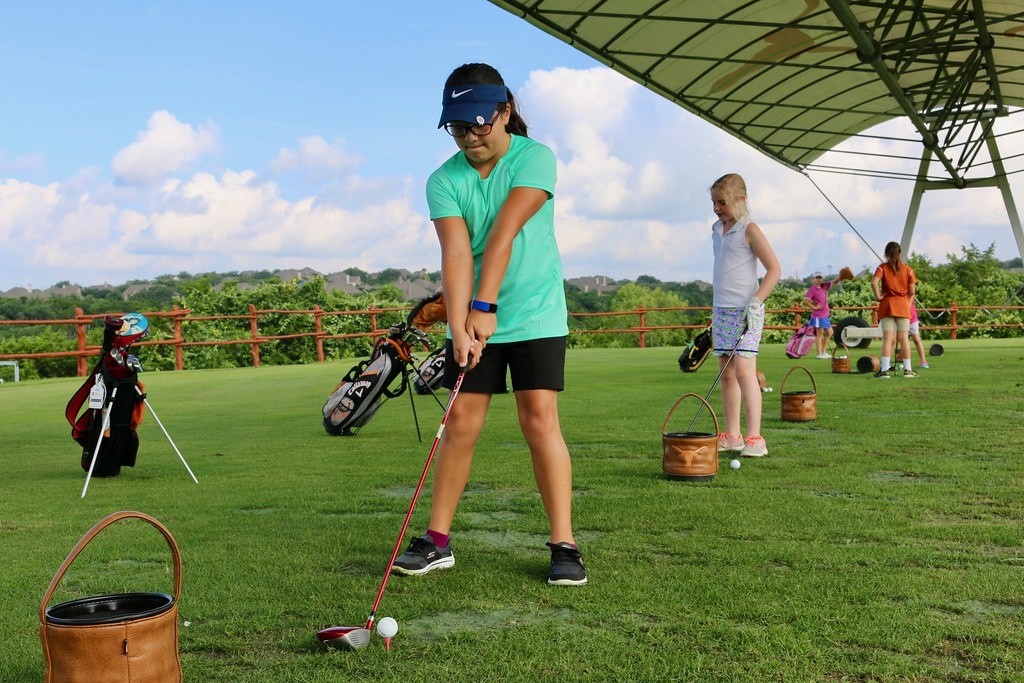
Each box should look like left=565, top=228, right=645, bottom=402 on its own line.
left=708, top=173, right=781, bottom=457
left=390, top=64, right=589, bottom=584
left=908, top=302, right=929, bottom=369
left=872, top=241, right=920, bottom=378
left=803, top=272, right=840, bottom=359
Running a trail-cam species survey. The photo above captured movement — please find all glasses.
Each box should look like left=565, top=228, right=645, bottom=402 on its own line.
left=444, top=108, right=502, bottom=136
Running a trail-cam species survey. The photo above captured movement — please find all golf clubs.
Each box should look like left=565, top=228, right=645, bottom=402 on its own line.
left=687, top=324, right=749, bottom=432
left=403, top=328, right=432, bottom=364
left=110, top=347, right=144, bottom=373
left=316, top=351, right=475, bottom=652
left=894, top=329, right=899, bottom=375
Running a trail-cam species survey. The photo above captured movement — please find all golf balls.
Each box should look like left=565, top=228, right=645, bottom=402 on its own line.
left=761, top=386, right=773, bottom=392
left=899, top=365, right=903, bottom=368
left=375, top=617, right=400, bottom=638
left=730, top=459, right=740, bottom=469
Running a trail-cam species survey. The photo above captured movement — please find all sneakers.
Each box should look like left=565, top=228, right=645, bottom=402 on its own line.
left=823, top=353, right=832, bottom=358
left=717, top=433, right=745, bottom=451
left=740, top=436, right=768, bottom=456
left=546, top=542, right=587, bottom=585
left=874, top=368, right=890, bottom=378
left=816, top=355, right=829, bottom=358
left=904, top=369, right=920, bottom=377
left=391, top=534, right=454, bottom=575
left=921, top=362, right=929, bottom=368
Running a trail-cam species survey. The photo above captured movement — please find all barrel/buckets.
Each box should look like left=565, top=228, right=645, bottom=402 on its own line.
left=929, top=344, right=943, bottom=356
left=897, top=349, right=905, bottom=362
left=856, top=354, right=880, bottom=373
left=782, top=391, right=816, bottom=423
left=45, top=591, right=174, bottom=625
left=755, top=370, right=767, bottom=388
left=833, top=356, right=849, bottom=374
left=663, top=432, right=716, bottom=483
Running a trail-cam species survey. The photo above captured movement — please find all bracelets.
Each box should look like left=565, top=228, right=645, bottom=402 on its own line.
left=470, top=298, right=497, bottom=313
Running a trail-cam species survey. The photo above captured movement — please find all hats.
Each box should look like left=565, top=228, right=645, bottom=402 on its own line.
left=813, top=271, right=824, bottom=278
left=437, top=85, right=507, bottom=128
